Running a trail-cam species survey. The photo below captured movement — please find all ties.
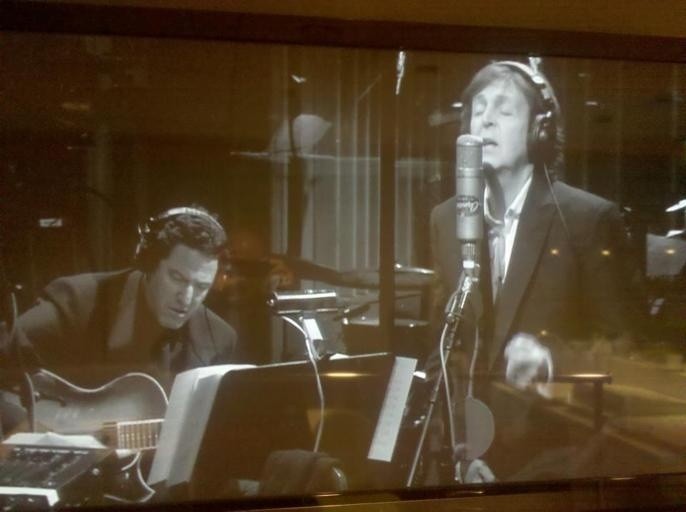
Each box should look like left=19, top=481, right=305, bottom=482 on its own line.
left=152, top=340, right=171, bottom=380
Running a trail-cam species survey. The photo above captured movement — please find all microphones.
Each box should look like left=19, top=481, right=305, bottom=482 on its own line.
left=454, top=133, right=486, bottom=283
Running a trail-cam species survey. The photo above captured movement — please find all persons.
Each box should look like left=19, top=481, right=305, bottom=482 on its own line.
left=0, top=204, right=258, bottom=456
left=425, top=59, right=642, bottom=486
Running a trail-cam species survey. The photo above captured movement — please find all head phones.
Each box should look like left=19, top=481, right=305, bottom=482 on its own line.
left=491, top=59, right=559, bottom=165
left=132, top=207, right=232, bottom=288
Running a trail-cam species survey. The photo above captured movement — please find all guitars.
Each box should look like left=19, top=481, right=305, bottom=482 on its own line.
left=8, top=368, right=170, bottom=465
